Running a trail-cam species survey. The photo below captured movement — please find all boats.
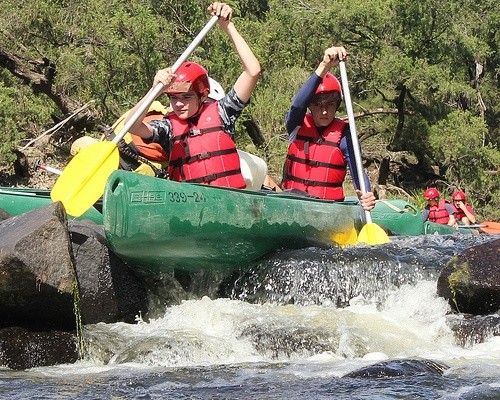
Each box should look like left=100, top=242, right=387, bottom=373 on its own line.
left=0, top=169, right=500, bottom=275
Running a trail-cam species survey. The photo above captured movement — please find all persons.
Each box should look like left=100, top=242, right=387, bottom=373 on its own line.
left=422, top=188, right=456, bottom=226
left=124, top=2, right=261, bottom=187
left=450, top=191, right=476, bottom=226
left=263, top=46, right=376, bottom=210
left=101, top=76, right=225, bottom=177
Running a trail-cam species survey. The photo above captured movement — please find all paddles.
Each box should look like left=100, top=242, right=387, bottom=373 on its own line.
left=50, top=13, right=229, bottom=218
left=458, top=221, right=500, bottom=234
left=338, top=54, right=391, bottom=244
left=33, top=160, right=63, bottom=175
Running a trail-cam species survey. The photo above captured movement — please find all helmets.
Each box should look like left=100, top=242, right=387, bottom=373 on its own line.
left=162, top=61, right=209, bottom=96
left=452, top=192, right=465, bottom=202
left=207, top=76, right=225, bottom=100
left=313, top=73, right=343, bottom=103
left=423, top=188, right=439, bottom=201
